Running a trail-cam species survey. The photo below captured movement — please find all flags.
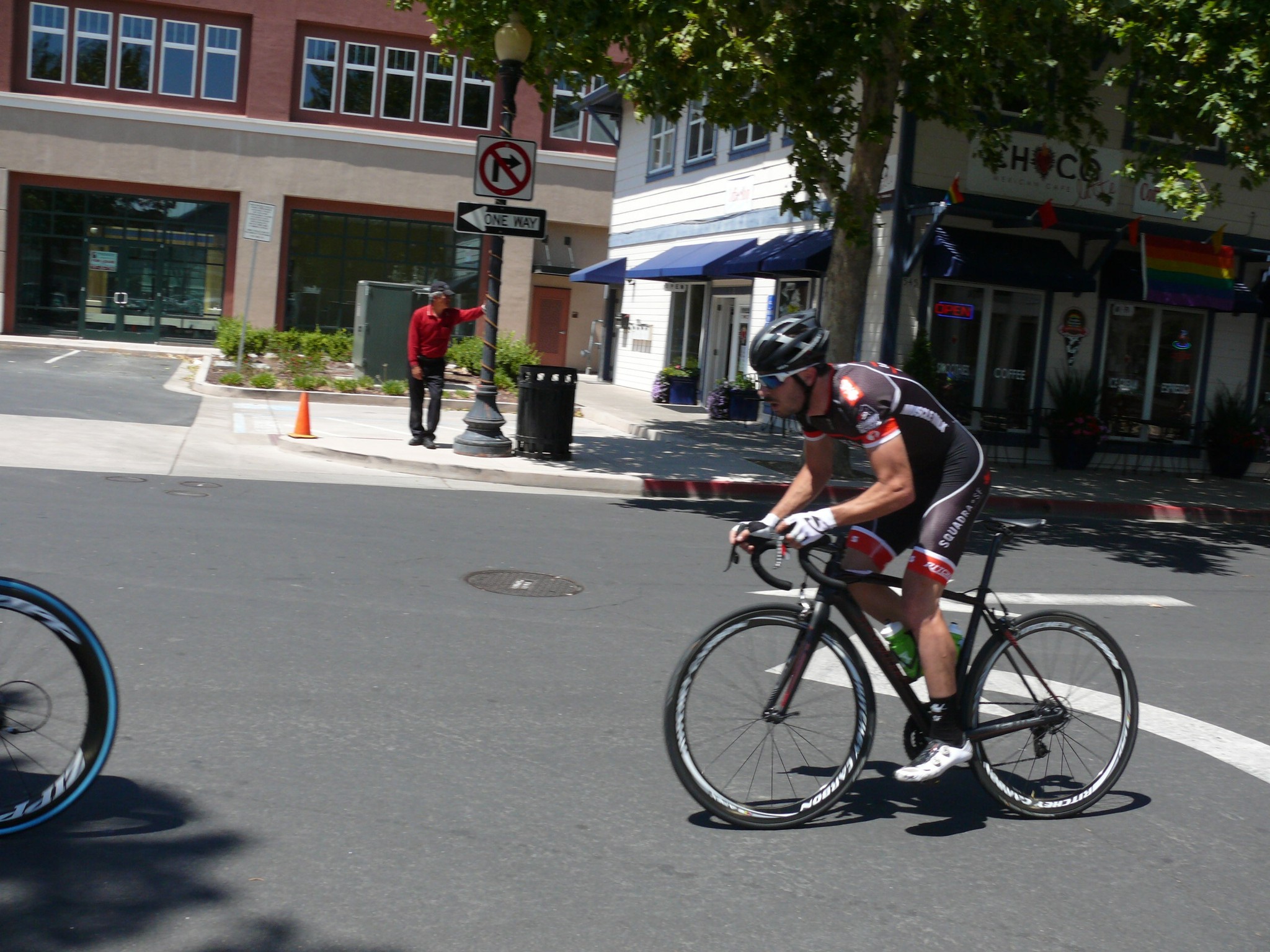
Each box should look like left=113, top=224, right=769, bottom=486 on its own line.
left=1039, top=198, right=1057, bottom=230
left=1140, top=232, right=1234, bottom=311
left=943, top=175, right=964, bottom=206
left=1128, top=216, right=1143, bottom=246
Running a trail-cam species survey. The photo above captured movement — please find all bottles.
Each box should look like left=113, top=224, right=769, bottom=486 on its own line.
left=880, top=619, right=924, bottom=679
left=946, top=621, right=964, bottom=662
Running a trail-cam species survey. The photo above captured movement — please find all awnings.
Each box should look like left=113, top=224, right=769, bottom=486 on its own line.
left=623, top=229, right=838, bottom=282
left=569, top=256, right=626, bottom=286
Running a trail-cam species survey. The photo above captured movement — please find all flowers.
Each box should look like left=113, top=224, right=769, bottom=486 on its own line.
left=1192, top=375, right=1270, bottom=456
left=1042, top=362, right=1110, bottom=445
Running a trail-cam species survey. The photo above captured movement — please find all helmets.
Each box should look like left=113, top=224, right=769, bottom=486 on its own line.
left=749, top=308, right=831, bottom=376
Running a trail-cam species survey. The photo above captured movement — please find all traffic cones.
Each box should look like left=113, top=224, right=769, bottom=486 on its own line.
left=287, top=392, right=319, bottom=439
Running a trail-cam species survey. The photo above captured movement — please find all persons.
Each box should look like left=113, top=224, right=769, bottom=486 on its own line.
left=730, top=309, right=992, bottom=782
left=406, top=281, right=487, bottom=449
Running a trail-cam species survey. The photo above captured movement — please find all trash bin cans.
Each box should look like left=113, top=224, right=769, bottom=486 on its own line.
left=516, top=364, right=577, bottom=460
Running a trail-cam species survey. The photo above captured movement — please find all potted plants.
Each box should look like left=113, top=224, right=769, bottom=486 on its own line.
left=720, top=373, right=761, bottom=422
left=659, top=365, right=700, bottom=406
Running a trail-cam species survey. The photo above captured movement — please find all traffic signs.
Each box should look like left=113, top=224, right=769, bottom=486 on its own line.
left=454, top=200, right=548, bottom=239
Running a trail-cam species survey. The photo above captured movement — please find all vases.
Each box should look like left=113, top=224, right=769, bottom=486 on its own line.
left=1048, top=431, right=1099, bottom=470
left=1204, top=442, right=1256, bottom=480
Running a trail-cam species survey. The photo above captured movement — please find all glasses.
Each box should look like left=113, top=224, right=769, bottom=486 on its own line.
left=757, top=362, right=822, bottom=389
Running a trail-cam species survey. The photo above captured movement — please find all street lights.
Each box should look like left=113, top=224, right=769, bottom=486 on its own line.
left=453, top=14, right=532, bottom=457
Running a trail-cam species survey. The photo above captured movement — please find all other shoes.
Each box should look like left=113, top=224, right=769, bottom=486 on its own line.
left=423, top=437, right=435, bottom=448
left=409, top=436, right=422, bottom=445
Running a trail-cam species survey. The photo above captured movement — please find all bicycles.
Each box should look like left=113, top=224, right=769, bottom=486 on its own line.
left=0, top=574, right=121, bottom=837
left=662, top=532, right=1140, bottom=832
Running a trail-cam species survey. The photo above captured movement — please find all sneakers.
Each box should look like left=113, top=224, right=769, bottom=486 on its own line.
left=894, top=736, right=972, bottom=782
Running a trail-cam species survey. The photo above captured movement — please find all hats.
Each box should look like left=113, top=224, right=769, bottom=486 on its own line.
left=430, top=281, right=456, bottom=296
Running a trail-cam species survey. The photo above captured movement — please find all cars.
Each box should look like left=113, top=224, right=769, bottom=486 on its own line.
left=108, top=294, right=222, bottom=315
